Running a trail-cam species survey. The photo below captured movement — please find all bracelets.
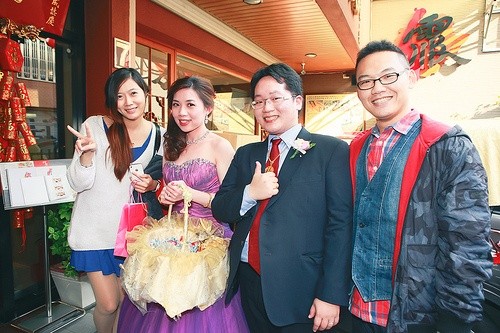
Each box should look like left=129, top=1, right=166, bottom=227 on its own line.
left=202, top=193, right=212, bottom=207
left=152, top=180, right=160, bottom=192
left=80, top=160, right=93, bottom=168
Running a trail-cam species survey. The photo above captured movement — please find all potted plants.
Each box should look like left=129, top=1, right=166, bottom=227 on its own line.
left=47, top=202, right=96, bottom=308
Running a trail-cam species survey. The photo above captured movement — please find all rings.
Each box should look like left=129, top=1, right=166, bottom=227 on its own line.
left=158, top=196, right=165, bottom=203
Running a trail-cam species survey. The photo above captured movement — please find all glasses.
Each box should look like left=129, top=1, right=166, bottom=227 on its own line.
left=250, top=95, right=296, bottom=109
left=355, top=68, right=407, bottom=90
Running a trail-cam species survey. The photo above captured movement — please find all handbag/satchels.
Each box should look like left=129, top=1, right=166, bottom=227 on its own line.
left=131, top=122, right=164, bottom=221
left=113, top=187, right=151, bottom=258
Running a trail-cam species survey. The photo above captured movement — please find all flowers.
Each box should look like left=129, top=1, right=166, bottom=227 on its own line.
left=289, top=138, right=316, bottom=159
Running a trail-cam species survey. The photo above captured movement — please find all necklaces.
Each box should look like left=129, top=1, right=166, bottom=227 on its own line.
left=265, top=146, right=288, bottom=173
left=187, top=129, right=210, bottom=145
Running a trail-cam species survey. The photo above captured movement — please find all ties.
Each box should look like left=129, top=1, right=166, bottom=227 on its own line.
left=248, top=139, right=281, bottom=275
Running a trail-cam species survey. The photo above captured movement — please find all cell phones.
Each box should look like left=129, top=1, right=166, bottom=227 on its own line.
left=129, top=163, right=144, bottom=179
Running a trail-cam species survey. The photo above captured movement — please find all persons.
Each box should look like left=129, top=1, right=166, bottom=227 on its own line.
left=349, top=40, right=494, bottom=333
left=117, top=76, right=249, bottom=333
left=211, top=62, right=353, bottom=333
left=67, top=68, right=167, bottom=333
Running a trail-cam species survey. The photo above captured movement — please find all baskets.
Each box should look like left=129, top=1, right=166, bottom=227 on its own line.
left=119, top=182, right=230, bottom=318
